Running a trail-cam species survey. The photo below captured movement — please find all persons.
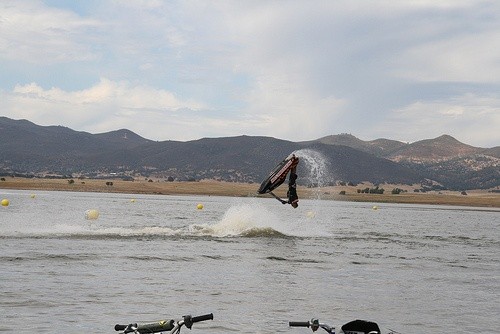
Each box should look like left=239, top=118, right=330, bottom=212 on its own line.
left=287, top=164, right=299, bottom=208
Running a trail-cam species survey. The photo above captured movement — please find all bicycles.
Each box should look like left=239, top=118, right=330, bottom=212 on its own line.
left=114, top=313, right=214, bottom=334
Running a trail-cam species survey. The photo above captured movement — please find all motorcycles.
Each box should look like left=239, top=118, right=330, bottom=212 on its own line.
left=257, top=154, right=299, bottom=204
